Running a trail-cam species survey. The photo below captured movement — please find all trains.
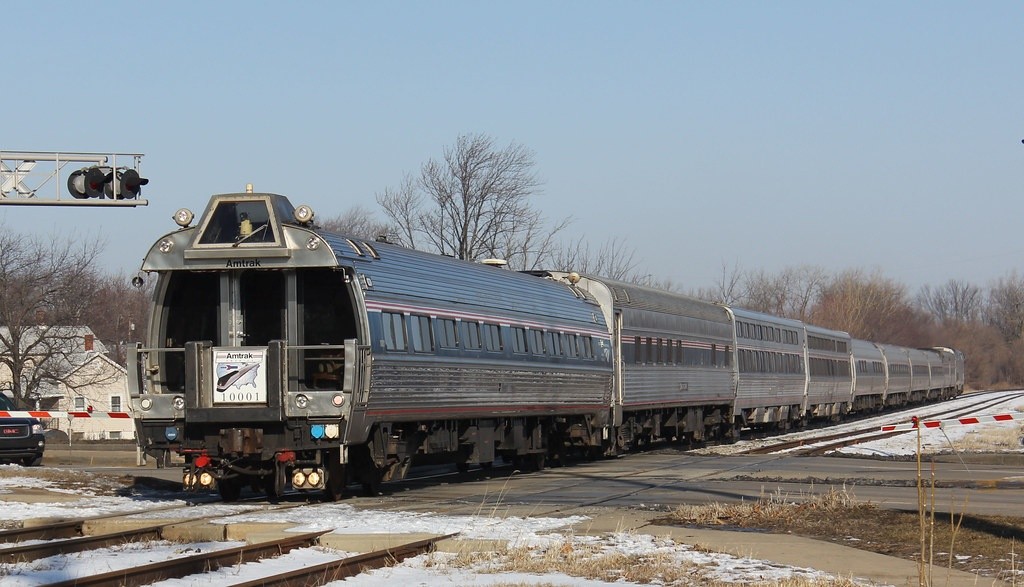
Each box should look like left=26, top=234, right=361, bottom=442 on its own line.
left=125, top=182, right=964, bottom=505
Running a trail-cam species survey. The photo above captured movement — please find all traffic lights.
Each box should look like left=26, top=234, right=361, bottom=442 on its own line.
left=70, top=164, right=147, bottom=199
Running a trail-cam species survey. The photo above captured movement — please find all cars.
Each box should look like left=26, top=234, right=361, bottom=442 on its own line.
left=0, top=393, right=46, bottom=466
